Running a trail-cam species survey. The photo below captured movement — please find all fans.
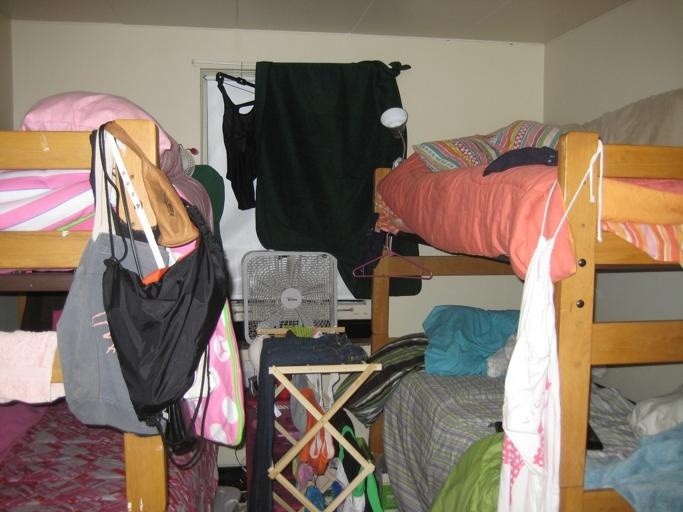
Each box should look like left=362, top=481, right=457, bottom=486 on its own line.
left=241, top=250, right=337, bottom=345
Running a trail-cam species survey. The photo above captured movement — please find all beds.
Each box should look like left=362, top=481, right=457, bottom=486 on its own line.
left=365, top=130, right=683, bottom=512
left=0, top=120, right=221, bottom=512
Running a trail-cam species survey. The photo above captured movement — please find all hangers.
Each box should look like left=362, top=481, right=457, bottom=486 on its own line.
left=351, top=232, right=432, bottom=280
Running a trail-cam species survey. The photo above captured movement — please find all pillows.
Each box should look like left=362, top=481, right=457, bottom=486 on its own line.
left=412, top=120, right=565, bottom=172
left=422, top=305, right=519, bottom=376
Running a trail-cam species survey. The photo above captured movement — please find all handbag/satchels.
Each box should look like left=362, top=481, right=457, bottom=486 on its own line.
left=56, top=232, right=247, bottom=448
